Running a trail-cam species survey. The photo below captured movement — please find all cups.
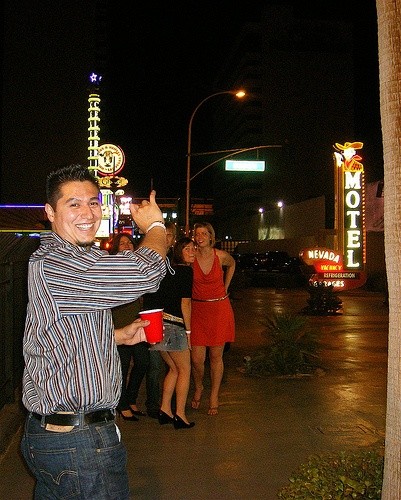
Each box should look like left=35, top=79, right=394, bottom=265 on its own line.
left=139, top=309, right=164, bottom=343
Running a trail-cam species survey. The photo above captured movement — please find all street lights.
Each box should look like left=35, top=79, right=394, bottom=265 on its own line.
left=185, top=89, right=245, bottom=238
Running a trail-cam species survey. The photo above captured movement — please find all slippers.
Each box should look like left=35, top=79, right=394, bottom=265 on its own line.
left=191, top=385, right=204, bottom=410
left=207, top=406, right=219, bottom=416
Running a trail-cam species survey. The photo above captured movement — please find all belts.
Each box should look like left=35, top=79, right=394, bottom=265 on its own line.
left=162, top=312, right=185, bottom=327
left=32, top=408, right=115, bottom=426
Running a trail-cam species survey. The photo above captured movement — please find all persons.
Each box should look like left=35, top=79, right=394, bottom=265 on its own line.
left=19, top=164, right=168, bottom=500
left=109, top=222, right=236, bottom=430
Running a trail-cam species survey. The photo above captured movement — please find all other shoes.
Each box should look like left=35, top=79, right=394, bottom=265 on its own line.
left=147, top=408, right=176, bottom=415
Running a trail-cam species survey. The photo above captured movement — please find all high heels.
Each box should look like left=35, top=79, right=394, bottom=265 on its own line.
left=157, top=410, right=175, bottom=424
left=117, top=403, right=137, bottom=421
left=128, top=404, right=143, bottom=415
left=173, top=415, right=195, bottom=429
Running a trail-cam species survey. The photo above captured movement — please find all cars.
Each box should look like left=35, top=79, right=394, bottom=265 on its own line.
left=235, top=252, right=313, bottom=287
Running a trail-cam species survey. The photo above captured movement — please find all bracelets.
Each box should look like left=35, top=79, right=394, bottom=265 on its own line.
left=186, top=330, right=191, bottom=334
left=145, top=220, right=166, bottom=233
